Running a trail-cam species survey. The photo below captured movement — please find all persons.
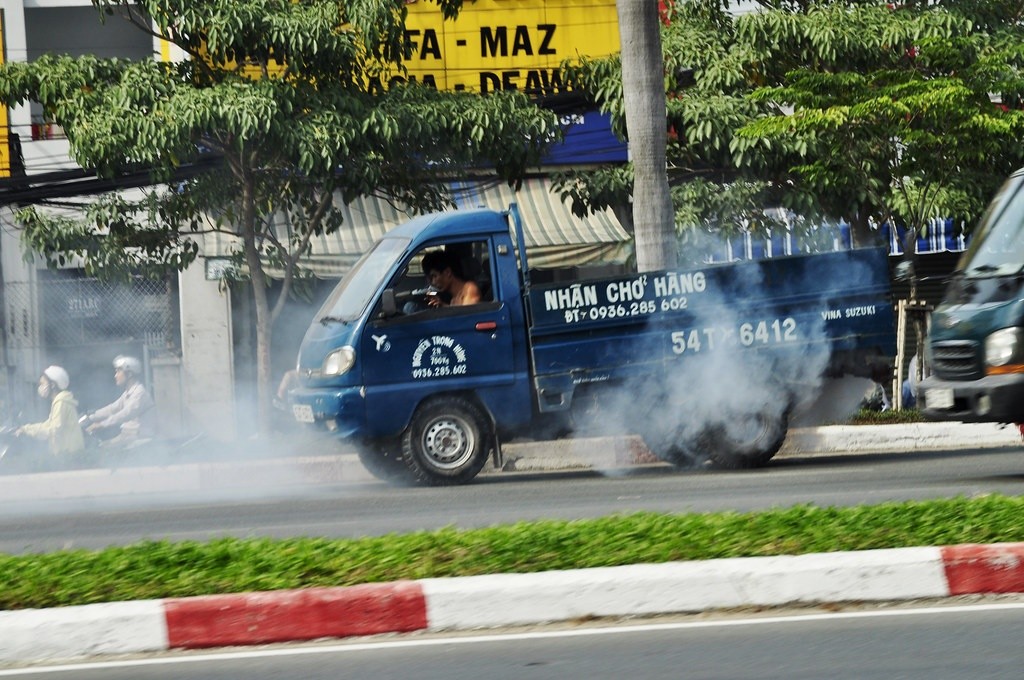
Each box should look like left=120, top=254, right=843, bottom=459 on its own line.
left=421, top=250, right=483, bottom=308
left=82, top=355, right=157, bottom=460
left=14, top=367, right=85, bottom=470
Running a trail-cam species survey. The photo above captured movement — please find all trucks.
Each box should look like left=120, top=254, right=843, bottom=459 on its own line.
left=287, top=199, right=899, bottom=487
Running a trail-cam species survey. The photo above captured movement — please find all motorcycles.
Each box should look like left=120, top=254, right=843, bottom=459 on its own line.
left=1, top=409, right=207, bottom=478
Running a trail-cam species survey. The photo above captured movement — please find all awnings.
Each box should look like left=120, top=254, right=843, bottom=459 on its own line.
left=690, top=206, right=978, bottom=268
left=188, top=187, right=635, bottom=282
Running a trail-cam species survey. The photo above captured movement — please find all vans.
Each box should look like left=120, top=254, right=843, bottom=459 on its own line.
left=912, top=161, right=1024, bottom=431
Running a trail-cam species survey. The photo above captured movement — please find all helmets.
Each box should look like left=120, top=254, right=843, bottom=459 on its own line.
left=45, top=365, right=70, bottom=391
left=112, top=357, right=139, bottom=374
